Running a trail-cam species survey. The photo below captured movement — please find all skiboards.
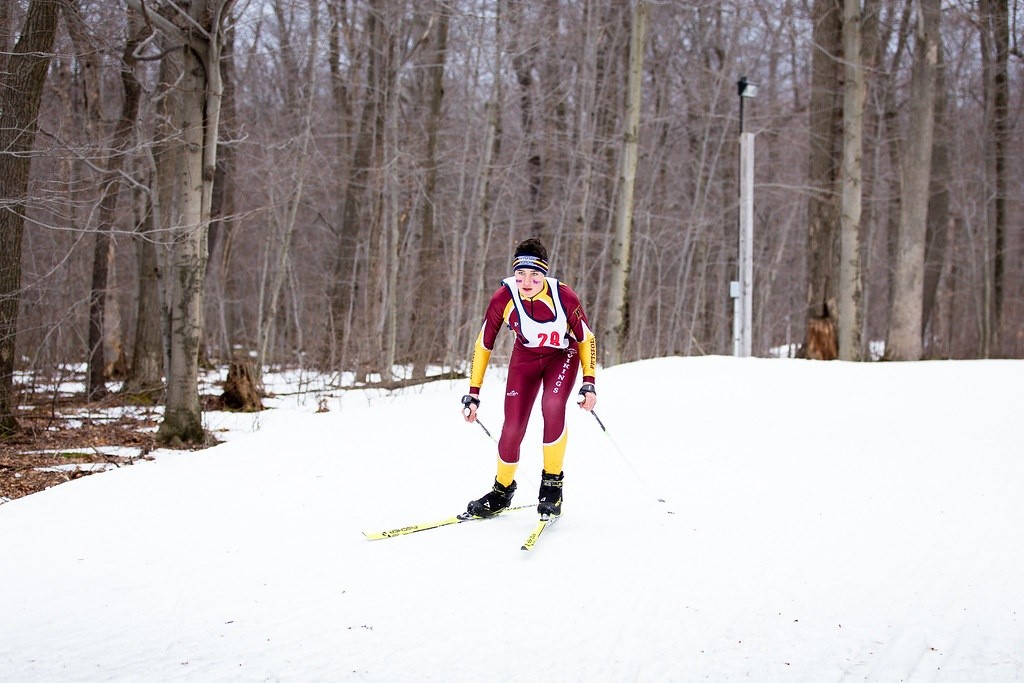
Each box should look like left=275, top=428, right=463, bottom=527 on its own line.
left=360, top=503, right=556, bottom=552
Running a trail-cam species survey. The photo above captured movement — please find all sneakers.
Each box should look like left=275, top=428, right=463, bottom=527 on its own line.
left=467, top=475, right=517, bottom=517
left=537, top=469, right=564, bottom=517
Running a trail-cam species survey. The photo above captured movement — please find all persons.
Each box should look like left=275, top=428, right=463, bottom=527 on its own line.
left=461, top=237, right=596, bottom=520
